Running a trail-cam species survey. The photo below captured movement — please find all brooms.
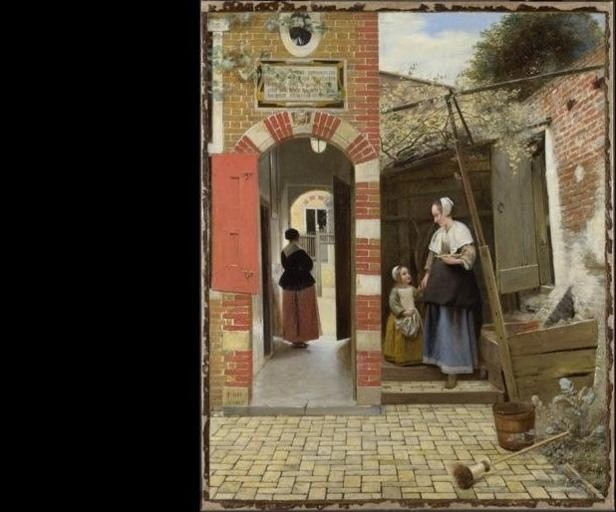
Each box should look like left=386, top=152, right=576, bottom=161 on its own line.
left=454, top=431, right=569, bottom=491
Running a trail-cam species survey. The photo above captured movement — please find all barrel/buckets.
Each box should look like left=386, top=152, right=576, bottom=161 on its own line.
left=492, top=400, right=536, bottom=451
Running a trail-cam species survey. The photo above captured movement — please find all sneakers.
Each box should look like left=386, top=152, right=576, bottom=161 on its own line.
left=446, top=372, right=457, bottom=388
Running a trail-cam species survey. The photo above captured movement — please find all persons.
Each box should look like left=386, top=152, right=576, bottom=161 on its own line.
left=418, top=196, right=483, bottom=390
left=275, top=228, right=323, bottom=350
left=381, top=264, right=424, bottom=368
